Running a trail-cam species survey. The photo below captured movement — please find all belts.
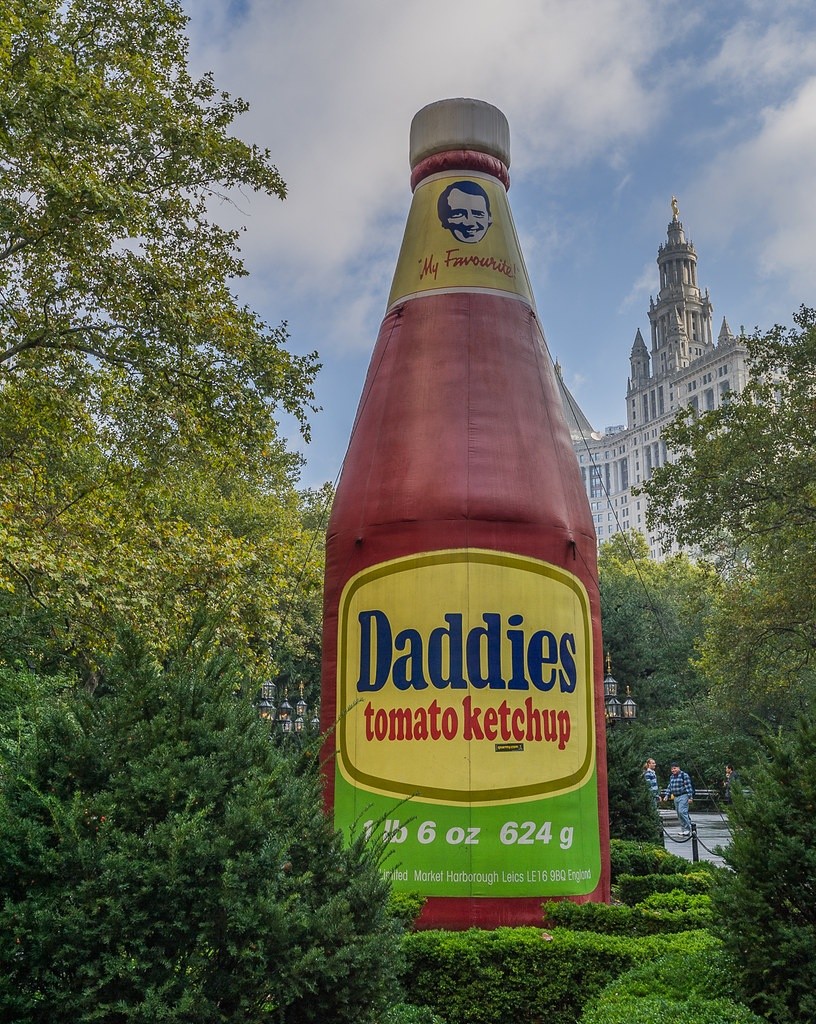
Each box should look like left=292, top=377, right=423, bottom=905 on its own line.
left=673, top=793, right=688, bottom=797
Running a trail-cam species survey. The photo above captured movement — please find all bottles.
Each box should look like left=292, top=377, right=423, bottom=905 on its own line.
left=317, top=95, right=609, bottom=934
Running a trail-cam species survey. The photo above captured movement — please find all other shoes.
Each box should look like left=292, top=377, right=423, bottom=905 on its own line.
left=682, top=831, right=690, bottom=836
left=678, top=831, right=684, bottom=835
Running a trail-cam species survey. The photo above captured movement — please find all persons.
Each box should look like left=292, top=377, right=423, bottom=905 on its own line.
left=721, top=765, right=735, bottom=805
left=644, top=758, right=662, bottom=807
left=663, top=761, right=693, bottom=835
